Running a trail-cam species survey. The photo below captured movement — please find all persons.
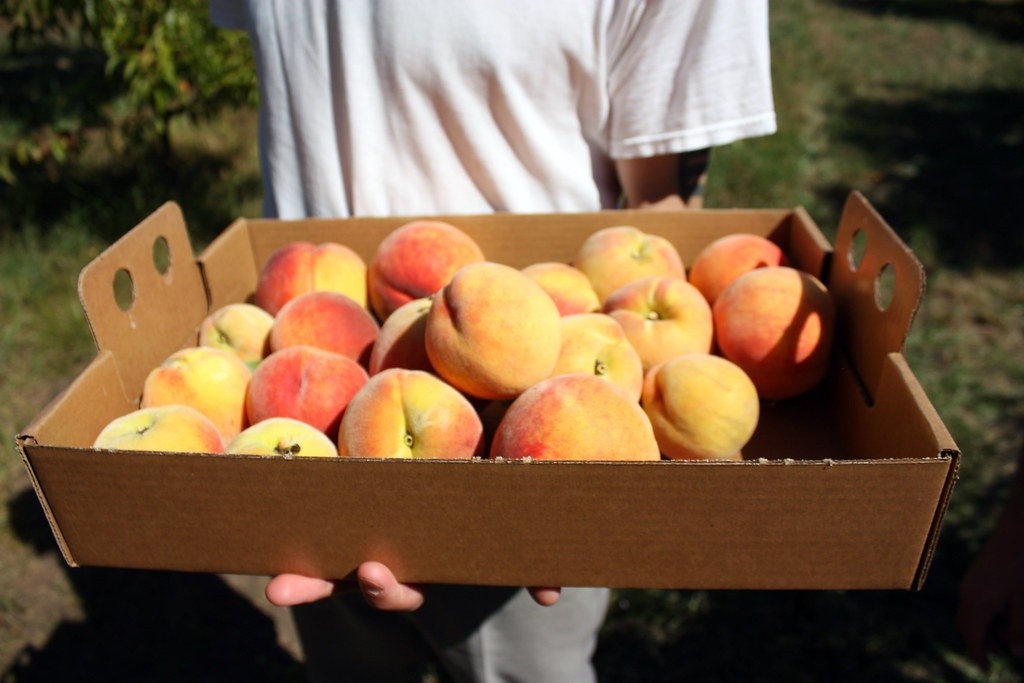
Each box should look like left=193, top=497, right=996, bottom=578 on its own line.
left=198, top=0, right=780, bottom=683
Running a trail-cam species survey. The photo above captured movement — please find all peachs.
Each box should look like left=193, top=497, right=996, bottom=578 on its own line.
left=90, top=219, right=836, bottom=462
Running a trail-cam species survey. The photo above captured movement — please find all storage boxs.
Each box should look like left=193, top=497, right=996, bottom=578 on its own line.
left=15, top=186, right=963, bottom=594
